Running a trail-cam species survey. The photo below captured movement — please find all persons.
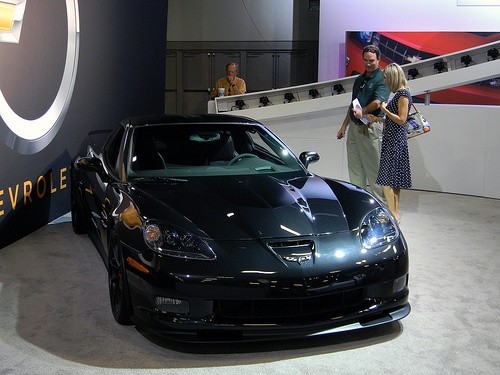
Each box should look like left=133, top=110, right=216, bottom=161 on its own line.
left=337, top=45, right=392, bottom=208
left=210, top=62, right=246, bottom=100
left=366, top=63, right=412, bottom=224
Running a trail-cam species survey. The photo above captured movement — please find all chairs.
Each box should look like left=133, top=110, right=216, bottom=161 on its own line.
left=203, top=131, right=240, bottom=167
left=132, top=130, right=164, bottom=170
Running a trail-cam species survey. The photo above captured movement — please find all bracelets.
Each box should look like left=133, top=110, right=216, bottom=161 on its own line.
left=232, top=85, right=235, bottom=87
left=384, top=110, right=389, bottom=115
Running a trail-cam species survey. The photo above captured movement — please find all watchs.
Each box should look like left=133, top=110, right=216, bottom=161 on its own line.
left=362, top=108, right=367, bottom=114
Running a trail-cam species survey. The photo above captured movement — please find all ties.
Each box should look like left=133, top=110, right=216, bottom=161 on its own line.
left=355, top=78, right=369, bottom=99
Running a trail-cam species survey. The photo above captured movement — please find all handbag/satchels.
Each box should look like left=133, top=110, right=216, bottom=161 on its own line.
left=406, top=103, right=431, bottom=139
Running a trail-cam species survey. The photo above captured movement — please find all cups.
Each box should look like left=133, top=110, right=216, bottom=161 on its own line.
left=217, top=87, right=225, bottom=97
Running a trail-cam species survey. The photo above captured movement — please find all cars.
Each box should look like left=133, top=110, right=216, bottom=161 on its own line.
left=69, top=113, right=411, bottom=346
left=345, top=31, right=500, bottom=105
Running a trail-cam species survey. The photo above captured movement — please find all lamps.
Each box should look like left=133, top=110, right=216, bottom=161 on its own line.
left=309, top=88, right=319, bottom=99
left=235, top=100, right=243, bottom=109
left=282, top=92, right=294, bottom=102
left=433, top=61, right=445, bottom=73
left=487, top=48, right=500, bottom=62
left=260, top=96, right=270, bottom=105
left=333, top=83, right=344, bottom=94
left=408, top=68, right=420, bottom=77
left=461, top=55, right=474, bottom=68
